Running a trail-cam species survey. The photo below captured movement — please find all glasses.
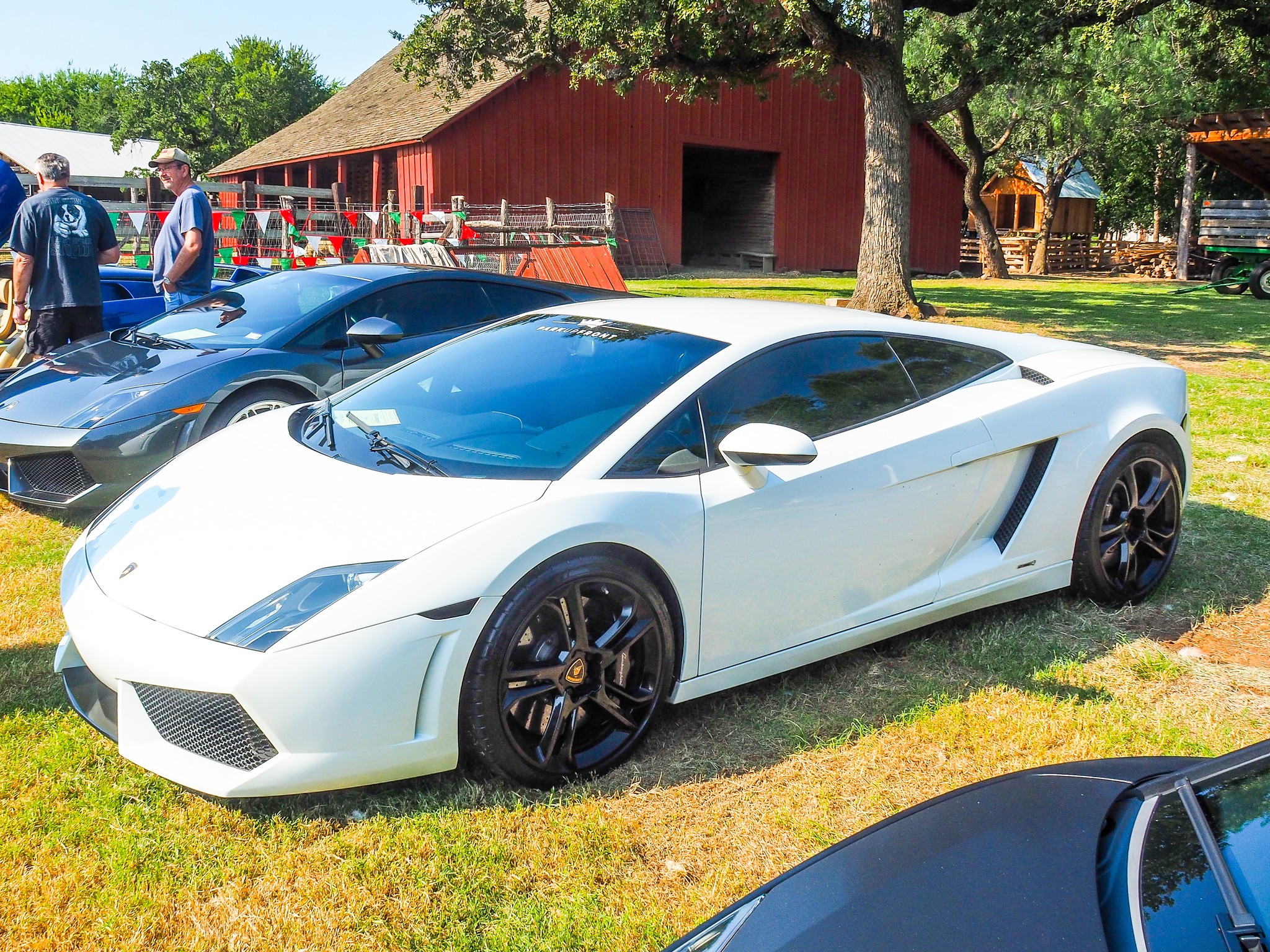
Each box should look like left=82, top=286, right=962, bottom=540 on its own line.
left=155, top=164, right=184, bottom=177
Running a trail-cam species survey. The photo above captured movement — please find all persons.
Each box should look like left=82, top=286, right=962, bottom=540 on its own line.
left=149, top=148, right=214, bottom=312
left=7, top=153, right=120, bottom=362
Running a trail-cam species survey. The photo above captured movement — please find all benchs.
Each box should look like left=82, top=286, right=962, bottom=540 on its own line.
left=739, top=251, right=777, bottom=273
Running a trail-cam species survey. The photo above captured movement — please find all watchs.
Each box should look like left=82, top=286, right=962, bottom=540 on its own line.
left=163, top=277, right=174, bottom=285
left=12, top=299, right=25, bottom=304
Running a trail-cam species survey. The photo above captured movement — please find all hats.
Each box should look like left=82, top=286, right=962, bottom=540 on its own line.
left=148, top=147, right=190, bottom=168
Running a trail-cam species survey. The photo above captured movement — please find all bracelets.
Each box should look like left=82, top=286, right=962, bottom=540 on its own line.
left=15, top=303, right=29, bottom=309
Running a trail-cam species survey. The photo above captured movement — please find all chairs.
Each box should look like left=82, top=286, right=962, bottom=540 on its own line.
left=708, top=361, right=873, bottom=449
left=0, top=278, right=31, bottom=369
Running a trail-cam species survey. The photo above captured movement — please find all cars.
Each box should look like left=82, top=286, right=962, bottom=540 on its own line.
left=659, top=733, right=1270, bottom=952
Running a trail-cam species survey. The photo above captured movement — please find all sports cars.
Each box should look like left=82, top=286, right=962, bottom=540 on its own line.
left=0, top=260, right=280, bottom=370
left=48, top=294, right=1191, bottom=804
left=0, top=258, right=653, bottom=521
